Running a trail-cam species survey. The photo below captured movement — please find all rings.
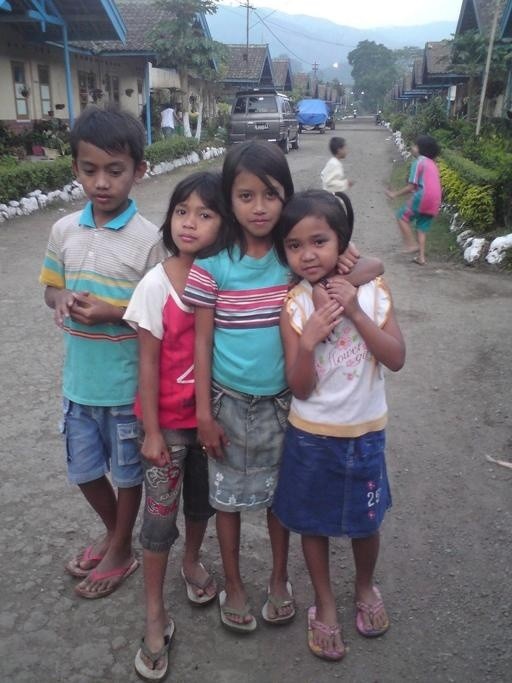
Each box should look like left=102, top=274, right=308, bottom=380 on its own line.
left=200, top=446, right=207, bottom=452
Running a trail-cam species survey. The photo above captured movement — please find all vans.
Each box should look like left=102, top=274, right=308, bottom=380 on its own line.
left=295, top=97, right=336, bottom=134
left=227, top=87, right=301, bottom=152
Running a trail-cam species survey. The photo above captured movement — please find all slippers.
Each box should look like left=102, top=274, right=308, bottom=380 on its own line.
left=75, top=558, right=140, bottom=599
left=217, top=588, right=258, bottom=635
left=134, top=614, right=175, bottom=682
left=305, top=604, right=346, bottom=661
left=66, top=544, right=104, bottom=576
left=402, top=242, right=425, bottom=265
left=354, top=583, right=390, bottom=638
left=261, top=580, right=295, bottom=624
left=180, top=560, right=218, bottom=607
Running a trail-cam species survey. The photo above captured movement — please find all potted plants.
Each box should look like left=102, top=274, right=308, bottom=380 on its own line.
left=40, top=130, right=63, bottom=157
left=30, top=130, right=45, bottom=155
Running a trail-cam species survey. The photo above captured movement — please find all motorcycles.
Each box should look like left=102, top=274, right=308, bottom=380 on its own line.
left=376, top=112, right=383, bottom=125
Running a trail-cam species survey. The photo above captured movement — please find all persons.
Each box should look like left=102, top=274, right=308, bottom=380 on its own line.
left=121, top=169, right=359, bottom=683
left=352, top=108, right=356, bottom=118
left=383, top=135, right=442, bottom=264
left=273, top=188, right=407, bottom=661
left=189, top=138, right=384, bottom=636
left=158, top=103, right=181, bottom=140
left=38, top=108, right=173, bottom=598
left=322, top=134, right=353, bottom=196
